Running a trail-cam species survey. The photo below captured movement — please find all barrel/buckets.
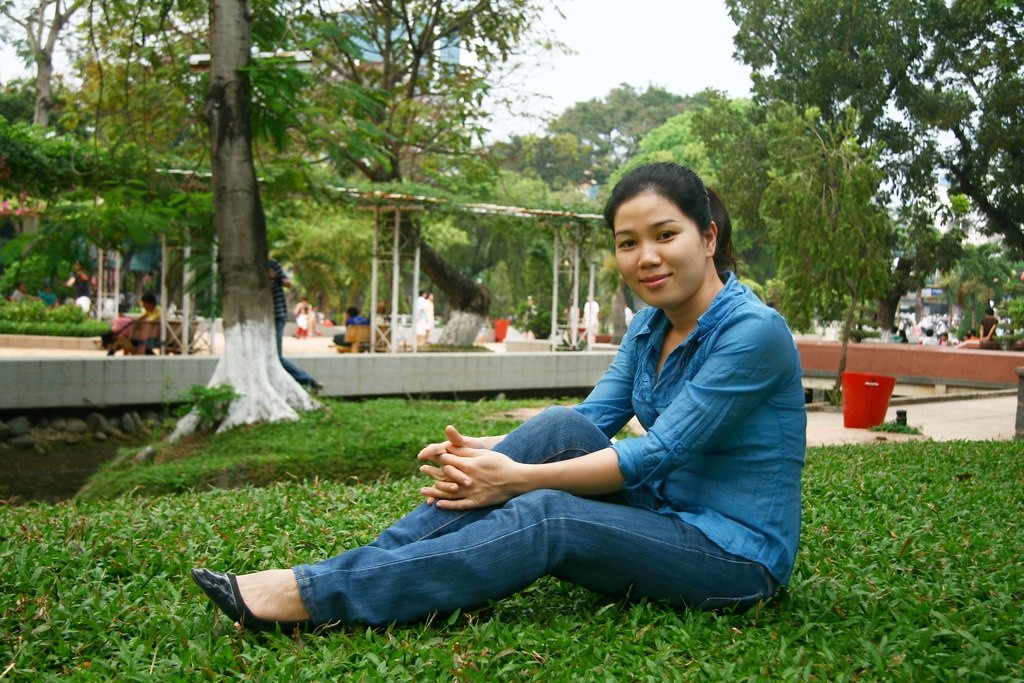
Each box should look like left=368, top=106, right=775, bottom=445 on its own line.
left=494, top=319, right=510, bottom=341
left=842, top=372, right=897, bottom=428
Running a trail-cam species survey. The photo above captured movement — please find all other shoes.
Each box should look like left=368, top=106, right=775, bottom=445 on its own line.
left=190, top=568, right=344, bottom=641
left=308, top=383, right=324, bottom=396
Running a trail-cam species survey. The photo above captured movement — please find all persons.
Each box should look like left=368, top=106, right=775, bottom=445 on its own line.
left=524, top=296, right=536, bottom=339
left=625, top=304, right=633, bottom=328
left=269, top=258, right=333, bottom=392
left=568, top=295, right=600, bottom=343
left=105, top=293, right=159, bottom=356
left=67, top=262, right=92, bottom=316
left=904, top=307, right=998, bottom=347
left=11, top=283, right=60, bottom=310
left=417, top=290, right=434, bottom=348
left=193, top=163, right=807, bottom=636
left=333, top=304, right=388, bottom=351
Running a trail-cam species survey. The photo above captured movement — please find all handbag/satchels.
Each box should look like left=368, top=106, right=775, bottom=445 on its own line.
left=100, top=330, right=116, bottom=348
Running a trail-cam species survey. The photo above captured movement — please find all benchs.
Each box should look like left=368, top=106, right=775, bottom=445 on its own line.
left=337, top=325, right=393, bottom=352
left=108, top=322, right=200, bottom=356
